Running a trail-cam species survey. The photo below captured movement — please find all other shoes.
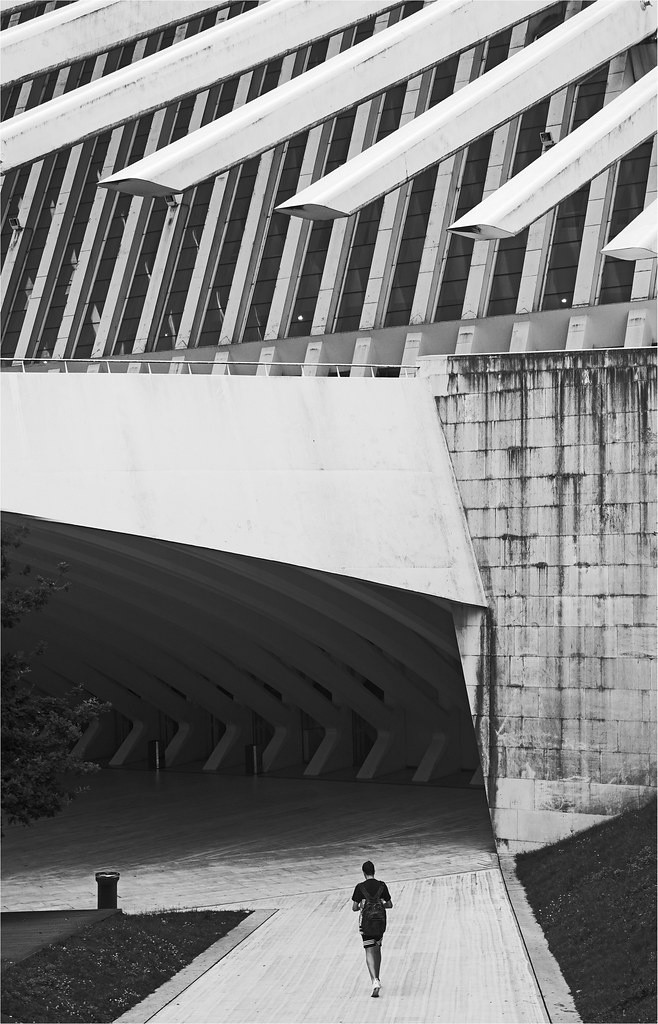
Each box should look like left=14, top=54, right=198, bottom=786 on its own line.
left=371, top=979, right=380, bottom=997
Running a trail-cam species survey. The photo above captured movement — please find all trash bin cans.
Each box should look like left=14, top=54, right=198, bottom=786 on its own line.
left=244, top=744, right=263, bottom=774
left=149, top=740, right=164, bottom=769
left=95, top=872, right=121, bottom=909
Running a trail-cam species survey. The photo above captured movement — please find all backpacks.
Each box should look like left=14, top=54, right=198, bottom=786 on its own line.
left=359, top=881, right=386, bottom=929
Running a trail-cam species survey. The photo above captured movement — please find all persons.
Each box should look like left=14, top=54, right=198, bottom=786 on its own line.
left=351, top=861, right=393, bottom=998
